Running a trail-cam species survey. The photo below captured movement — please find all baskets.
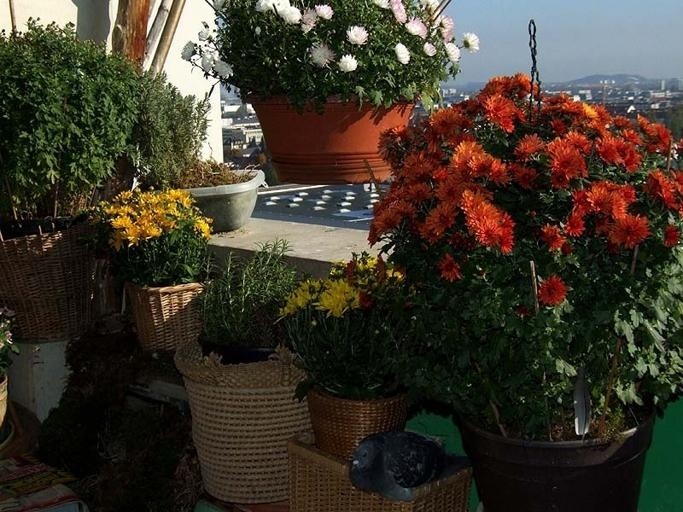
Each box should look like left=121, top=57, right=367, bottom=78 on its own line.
left=128, top=281, right=205, bottom=351
left=174, top=343, right=312, bottom=503
left=287, top=439, right=469, bottom=512
left=174, top=343, right=469, bottom=512
left=0, top=227, right=85, bottom=342
left=306, top=388, right=412, bottom=463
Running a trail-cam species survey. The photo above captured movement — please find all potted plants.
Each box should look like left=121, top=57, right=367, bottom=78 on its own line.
left=0, top=304, right=21, bottom=431
left=0, top=16, right=140, bottom=234
left=173, top=240, right=313, bottom=363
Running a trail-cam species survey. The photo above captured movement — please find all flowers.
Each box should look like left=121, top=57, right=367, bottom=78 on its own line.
left=275, top=251, right=420, bottom=401
left=180, top=0, right=481, bottom=113
left=366, top=71, right=680, bottom=438
left=94, top=186, right=219, bottom=287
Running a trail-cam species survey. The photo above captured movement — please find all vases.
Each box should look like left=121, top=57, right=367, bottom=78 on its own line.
left=458, top=414, right=656, bottom=509
left=189, top=169, right=265, bottom=230
left=252, top=95, right=417, bottom=182
